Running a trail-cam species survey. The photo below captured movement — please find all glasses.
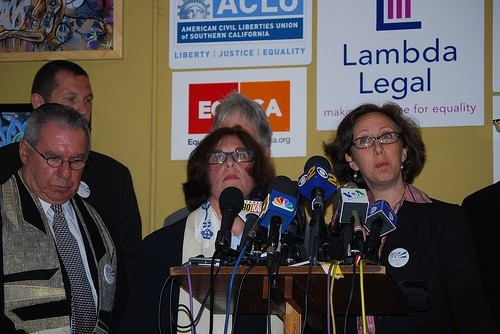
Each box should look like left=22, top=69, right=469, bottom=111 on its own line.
left=348, top=130, right=402, bottom=150
left=204, top=148, right=255, bottom=165
left=24, top=138, right=88, bottom=171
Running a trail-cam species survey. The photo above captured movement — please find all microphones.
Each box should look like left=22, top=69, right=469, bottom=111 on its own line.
left=215, top=187, right=245, bottom=257
left=298, top=155, right=337, bottom=220
left=239, top=175, right=398, bottom=266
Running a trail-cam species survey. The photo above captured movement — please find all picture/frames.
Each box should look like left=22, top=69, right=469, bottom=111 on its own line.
left=0, top=0, right=124, bottom=61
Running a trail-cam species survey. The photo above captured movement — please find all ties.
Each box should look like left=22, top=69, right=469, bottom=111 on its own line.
left=51, top=202, right=99, bottom=333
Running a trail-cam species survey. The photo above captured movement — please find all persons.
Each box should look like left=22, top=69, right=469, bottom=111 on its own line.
left=0, top=59, right=142, bottom=298
left=0, top=102, right=125, bottom=334
left=160, top=92, right=272, bottom=226
left=302, top=103, right=493, bottom=334
left=121, top=125, right=274, bottom=334
left=459, top=182, right=500, bottom=334
